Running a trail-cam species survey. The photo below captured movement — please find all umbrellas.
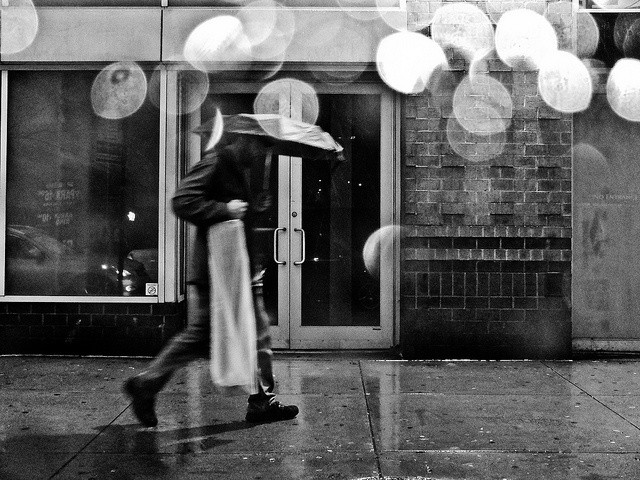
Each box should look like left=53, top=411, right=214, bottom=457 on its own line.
left=190, top=113, right=347, bottom=208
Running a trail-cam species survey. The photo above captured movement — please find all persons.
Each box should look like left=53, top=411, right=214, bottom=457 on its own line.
left=123, top=133, right=299, bottom=429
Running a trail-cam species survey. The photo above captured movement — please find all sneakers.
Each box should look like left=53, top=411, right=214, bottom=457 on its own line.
left=245, top=401, right=299, bottom=423
left=124, top=380, right=158, bottom=424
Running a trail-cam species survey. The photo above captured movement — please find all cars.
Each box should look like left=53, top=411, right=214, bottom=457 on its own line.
left=5, top=225, right=144, bottom=296
